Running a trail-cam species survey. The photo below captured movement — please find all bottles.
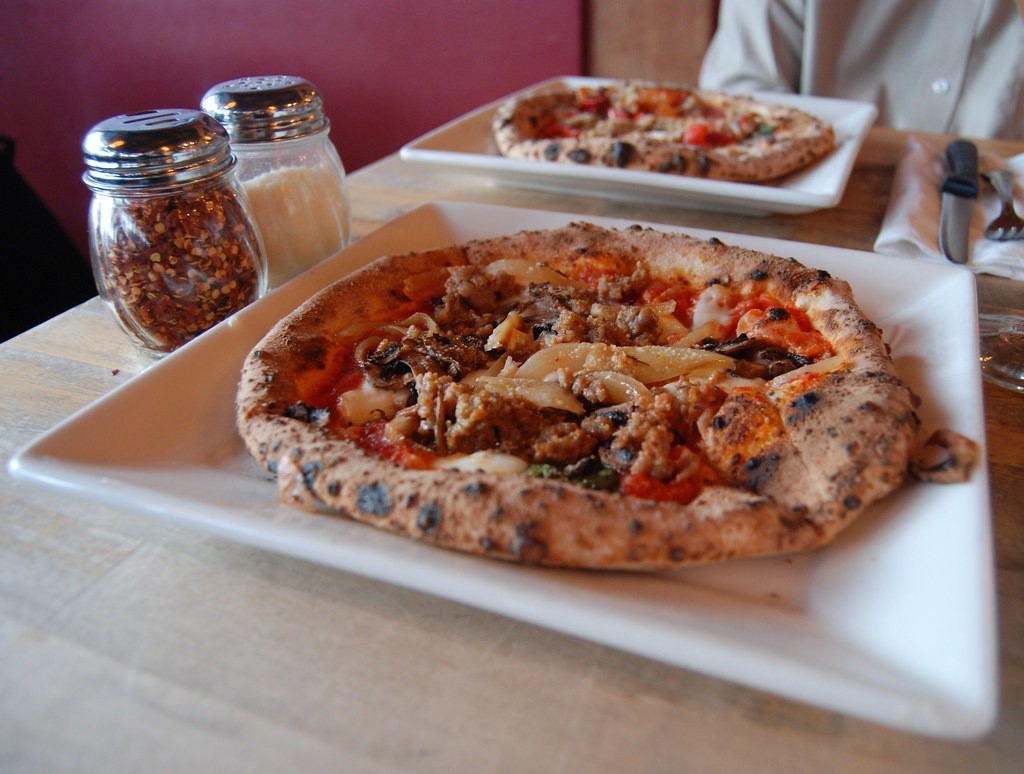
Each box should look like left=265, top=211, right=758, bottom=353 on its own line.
left=82, top=108, right=266, bottom=359
left=202, top=75, right=353, bottom=299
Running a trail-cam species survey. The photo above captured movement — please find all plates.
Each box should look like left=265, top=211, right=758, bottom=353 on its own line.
left=399, top=74, right=880, bottom=217
left=8, top=201, right=998, bottom=746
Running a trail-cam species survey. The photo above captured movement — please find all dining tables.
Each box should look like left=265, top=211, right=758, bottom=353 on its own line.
left=1, top=125, right=1023, bottom=773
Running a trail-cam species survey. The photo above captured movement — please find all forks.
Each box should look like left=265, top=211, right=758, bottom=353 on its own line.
left=982, top=168, right=1024, bottom=243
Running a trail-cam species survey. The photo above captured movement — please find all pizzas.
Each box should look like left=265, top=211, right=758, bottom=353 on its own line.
left=237, top=221, right=923, bottom=574
left=492, top=84, right=834, bottom=183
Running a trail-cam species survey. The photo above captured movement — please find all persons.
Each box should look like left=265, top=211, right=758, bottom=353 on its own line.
left=698, top=0, right=1023, bottom=143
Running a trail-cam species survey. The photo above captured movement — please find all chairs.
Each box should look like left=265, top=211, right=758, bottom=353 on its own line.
left=0, top=134, right=99, bottom=344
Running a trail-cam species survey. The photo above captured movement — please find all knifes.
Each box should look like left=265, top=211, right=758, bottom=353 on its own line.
left=937, top=138, right=979, bottom=265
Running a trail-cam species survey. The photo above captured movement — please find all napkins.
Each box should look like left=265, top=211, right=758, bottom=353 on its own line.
left=873, top=134, right=1024, bottom=280
